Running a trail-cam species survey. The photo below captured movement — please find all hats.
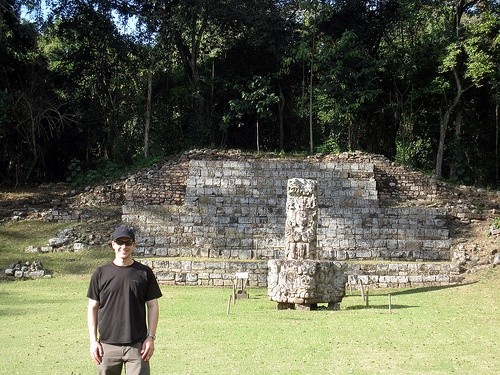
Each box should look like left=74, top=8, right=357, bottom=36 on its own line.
left=113, top=227, right=135, bottom=240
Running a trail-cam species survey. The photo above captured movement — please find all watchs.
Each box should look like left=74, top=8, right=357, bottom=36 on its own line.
left=148, top=334, right=156, bottom=340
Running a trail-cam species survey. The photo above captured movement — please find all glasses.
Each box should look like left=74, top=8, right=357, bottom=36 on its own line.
left=114, top=240, right=134, bottom=246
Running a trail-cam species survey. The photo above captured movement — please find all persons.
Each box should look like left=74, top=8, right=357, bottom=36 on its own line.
left=86, top=227, right=162, bottom=375
